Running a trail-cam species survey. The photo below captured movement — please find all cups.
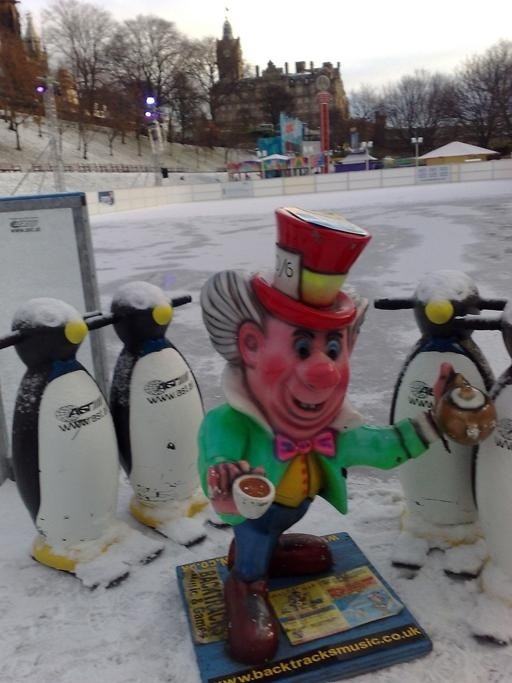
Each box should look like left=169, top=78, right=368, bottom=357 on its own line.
left=232, top=474, right=274, bottom=519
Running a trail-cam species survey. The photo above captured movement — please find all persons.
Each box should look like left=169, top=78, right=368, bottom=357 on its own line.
left=199, top=270, right=469, bottom=667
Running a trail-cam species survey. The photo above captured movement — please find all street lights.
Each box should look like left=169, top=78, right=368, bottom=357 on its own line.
left=411, top=136, right=424, bottom=167
left=361, top=141, right=373, bottom=170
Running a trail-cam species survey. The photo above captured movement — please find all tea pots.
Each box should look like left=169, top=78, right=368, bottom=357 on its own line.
left=437, top=371, right=496, bottom=447
